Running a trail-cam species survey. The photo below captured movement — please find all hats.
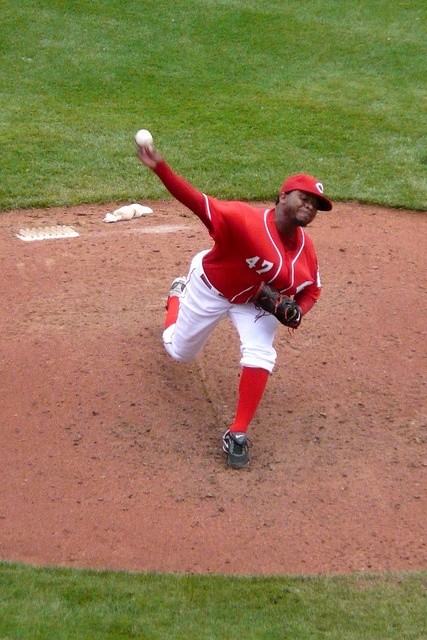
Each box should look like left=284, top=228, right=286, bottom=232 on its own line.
left=279, top=173, right=332, bottom=211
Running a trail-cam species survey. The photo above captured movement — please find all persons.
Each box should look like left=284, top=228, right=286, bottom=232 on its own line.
left=136, top=143, right=333, bottom=471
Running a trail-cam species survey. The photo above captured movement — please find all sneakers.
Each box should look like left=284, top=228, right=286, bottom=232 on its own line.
left=221, top=428, right=252, bottom=469
left=168, top=276, right=186, bottom=298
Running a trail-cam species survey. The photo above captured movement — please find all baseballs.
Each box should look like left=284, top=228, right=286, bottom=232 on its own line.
left=135, top=129, right=153, bottom=146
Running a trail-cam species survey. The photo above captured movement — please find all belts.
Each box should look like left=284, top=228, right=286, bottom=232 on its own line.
left=200, top=274, right=226, bottom=298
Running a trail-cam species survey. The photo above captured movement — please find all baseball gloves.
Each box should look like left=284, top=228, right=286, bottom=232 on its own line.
left=254, top=282, right=302, bottom=327
